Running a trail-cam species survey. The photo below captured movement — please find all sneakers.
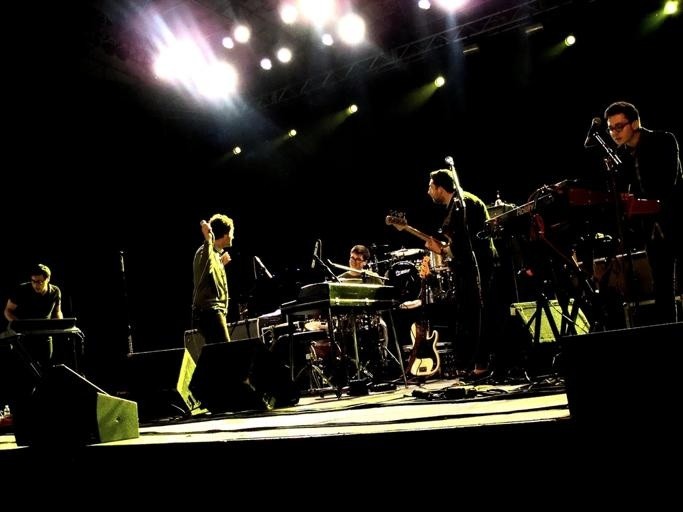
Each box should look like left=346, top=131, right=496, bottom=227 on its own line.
left=460, top=368, right=494, bottom=385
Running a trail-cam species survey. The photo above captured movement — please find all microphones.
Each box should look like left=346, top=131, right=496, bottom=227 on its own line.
left=119, top=251, right=125, bottom=272
left=310, top=241, right=319, bottom=269
left=584, top=117, right=601, bottom=147
left=255, top=256, right=273, bottom=279
left=444, top=155, right=454, bottom=166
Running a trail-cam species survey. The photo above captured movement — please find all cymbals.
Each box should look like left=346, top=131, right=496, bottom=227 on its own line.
left=387, top=248, right=423, bottom=258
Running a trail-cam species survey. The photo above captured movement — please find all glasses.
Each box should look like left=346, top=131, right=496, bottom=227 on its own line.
left=605, top=119, right=632, bottom=134
left=348, top=256, right=367, bottom=265
left=30, top=277, right=47, bottom=285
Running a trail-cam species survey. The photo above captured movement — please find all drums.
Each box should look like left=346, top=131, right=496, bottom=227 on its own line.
left=383, top=260, right=425, bottom=302
left=428, top=243, right=452, bottom=274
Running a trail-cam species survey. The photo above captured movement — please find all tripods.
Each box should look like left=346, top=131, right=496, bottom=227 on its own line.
left=295, top=344, right=341, bottom=398
left=443, top=169, right=533, bottom=386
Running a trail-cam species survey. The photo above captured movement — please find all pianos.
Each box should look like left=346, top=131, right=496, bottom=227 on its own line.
left=483, top=183, right=658, bottom=240
left=280, top=280, right=395, bottom=400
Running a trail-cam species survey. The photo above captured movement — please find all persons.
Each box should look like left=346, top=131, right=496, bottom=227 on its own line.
left=326, top=245, right=389, bottom=362
left=603, top=102, right=683, bottom=307
left=424, top=168, right=504, bottom=384
left=4, top=264, right=64, bottom=362
left=192, top=214, right=235, bottom=342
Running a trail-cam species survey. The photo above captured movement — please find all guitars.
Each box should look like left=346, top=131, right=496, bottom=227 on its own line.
left=409, top=258, right=440, bottom=375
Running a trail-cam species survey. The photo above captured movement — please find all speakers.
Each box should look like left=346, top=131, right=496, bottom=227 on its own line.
left=33, top=363, right=109, bottom=393
left=12, top=387, right=139, bottom=445
left=117, top=348, right=203, bottom=417
left=562, top=321, right=683, bottom=415
left=188, top=338, right=280, bottom=414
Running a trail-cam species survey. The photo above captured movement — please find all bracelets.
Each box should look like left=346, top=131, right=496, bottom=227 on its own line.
left=440, top=245, right=444, bottom=255
